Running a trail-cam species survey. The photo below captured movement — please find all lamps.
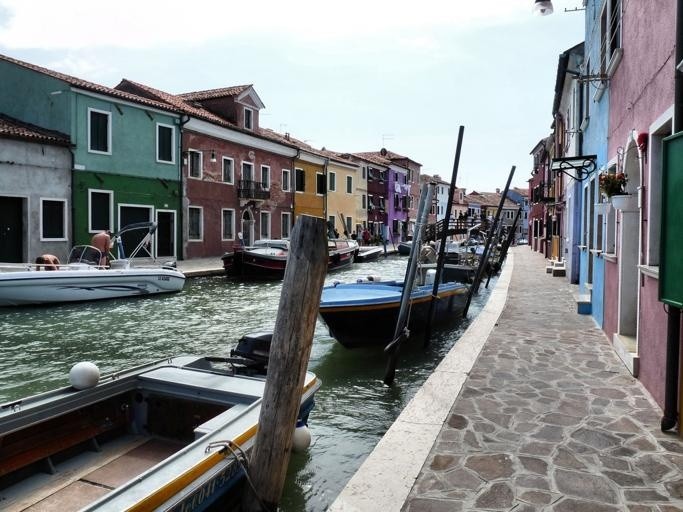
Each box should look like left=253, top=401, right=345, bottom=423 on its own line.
left=532, top=1, right=553, bottom=17
left=183, top=149, right=216, bottom=163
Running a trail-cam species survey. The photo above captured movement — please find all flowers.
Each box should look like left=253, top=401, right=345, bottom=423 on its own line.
left=597, top=172, right=628, bottom=196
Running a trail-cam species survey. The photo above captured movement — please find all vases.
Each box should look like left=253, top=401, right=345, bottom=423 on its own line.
left=594, top=203, right=610, bottom=215
left=611, top=195, right=631, bottom=210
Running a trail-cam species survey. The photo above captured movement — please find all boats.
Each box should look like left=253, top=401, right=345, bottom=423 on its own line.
left=318, top=277, right=472, bottom=350
left=0, top=332, right=324, bottom=512
left=0, top=222, right=187, bottom=308
left=414, top=243, right=483, bottom=283
left=221, top=238, right=382, bottom=279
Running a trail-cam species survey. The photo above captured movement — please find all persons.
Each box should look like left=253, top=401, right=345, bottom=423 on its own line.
left=35, top=254, right=60, bottom=271
left=458, top=210, right=487, bottom=229
left=237, top=230, right=245, bottom=246
left=90, top=230, right=111, bottom=265
left=342, top=225, right=374, bottom=246
